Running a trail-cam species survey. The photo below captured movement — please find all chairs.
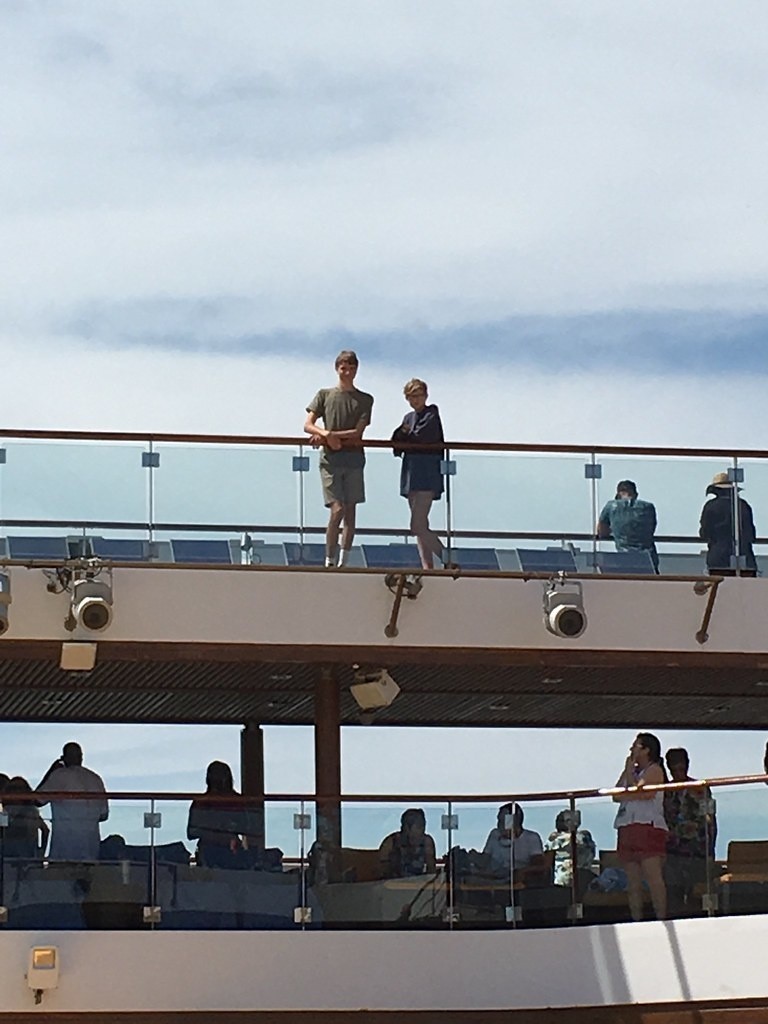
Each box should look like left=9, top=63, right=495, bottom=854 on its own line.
left=0, top=536, right=768, bottom=577
left=1, top=826, right=767, bottom=931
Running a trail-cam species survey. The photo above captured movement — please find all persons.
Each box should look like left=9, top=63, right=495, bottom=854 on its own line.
left=391, top=379, right=458, bottom=569
left=187, top=761, right=249, bottom=869
left=0, top=742, right=126, bottom=862
left=597, top=483, right=660, bottom=574
left=304, top=350, right=373, bottom=566
left=699, top=473, right=758, bottom=569
left=552, top=810, right=596, bottom=886
left=613, top=733, right=672, bottom=920
left=476, top=804, right=545, bottom=880
left=377, top=808, right=436, bottom=881
left=664, top=748, right=717, bottom=918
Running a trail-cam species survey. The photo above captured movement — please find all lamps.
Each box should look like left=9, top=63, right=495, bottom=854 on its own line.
left=694, top=579, right=725, bottom=642
left=58, top=643, right=99, bottom=670
left=384, top=571, right=423, bottom=636
left=0, top=574, right=13, bottom=637
left=44, top=563, right=118, bottom=632
left=351, top=665, right=401, bottom=710
left=538, top=570, right=589, bottom=639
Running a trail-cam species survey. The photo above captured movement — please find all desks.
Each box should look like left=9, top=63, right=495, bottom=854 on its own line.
left=383, top=882, right=526, bottom=924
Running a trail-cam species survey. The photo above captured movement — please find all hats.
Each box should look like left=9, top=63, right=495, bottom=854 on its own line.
left=615, top=480, right=638, bottom=500
left=706, top=473, right=745, bottom=496
